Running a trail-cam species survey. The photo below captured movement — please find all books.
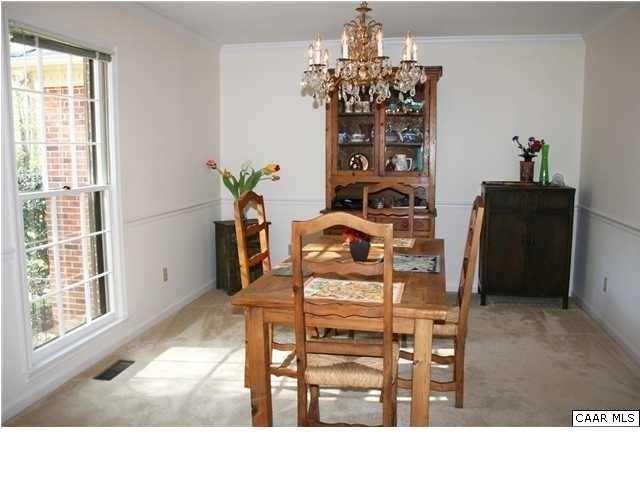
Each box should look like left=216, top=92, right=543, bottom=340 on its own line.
left=273, top=266, right=314, bottom=278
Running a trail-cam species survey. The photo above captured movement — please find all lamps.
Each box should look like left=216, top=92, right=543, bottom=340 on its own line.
left=300, top=1, right=427, bottom=105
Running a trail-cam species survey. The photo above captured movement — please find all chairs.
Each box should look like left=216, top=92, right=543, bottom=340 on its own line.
left=233, top=180, right=486, bottom=426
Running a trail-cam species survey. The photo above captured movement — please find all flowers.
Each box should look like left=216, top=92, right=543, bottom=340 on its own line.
left=512, top=135, right=545, bottom=162
left=207, top=158, right=280, bottom=199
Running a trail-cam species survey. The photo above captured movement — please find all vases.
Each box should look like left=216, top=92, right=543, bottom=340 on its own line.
left=520, top=161, right=535, bottom=182
left=540, top=145, right=549, bottom=183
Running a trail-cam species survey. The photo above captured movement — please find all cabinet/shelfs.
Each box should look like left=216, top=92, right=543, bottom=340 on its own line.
left=212, top=220, right=271, bottom=296
left=325, top=65, right=442, bottom=239
left=481, top=182, right=576, bottom=310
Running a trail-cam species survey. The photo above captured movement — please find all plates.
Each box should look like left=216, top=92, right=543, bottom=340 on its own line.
left=349, top=152, right=369, bottom=172
